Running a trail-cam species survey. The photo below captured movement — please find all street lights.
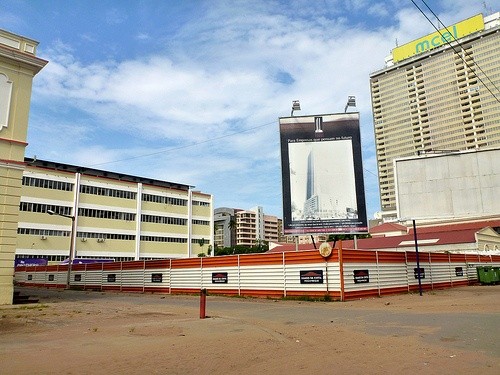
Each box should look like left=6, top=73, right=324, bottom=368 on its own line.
left=47, top=209, right=78, bottom=290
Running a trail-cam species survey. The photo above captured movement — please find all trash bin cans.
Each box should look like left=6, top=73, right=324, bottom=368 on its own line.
left=492, top=266, right=500, bottom=284
left=476, top=266, right=495, bottom=285
